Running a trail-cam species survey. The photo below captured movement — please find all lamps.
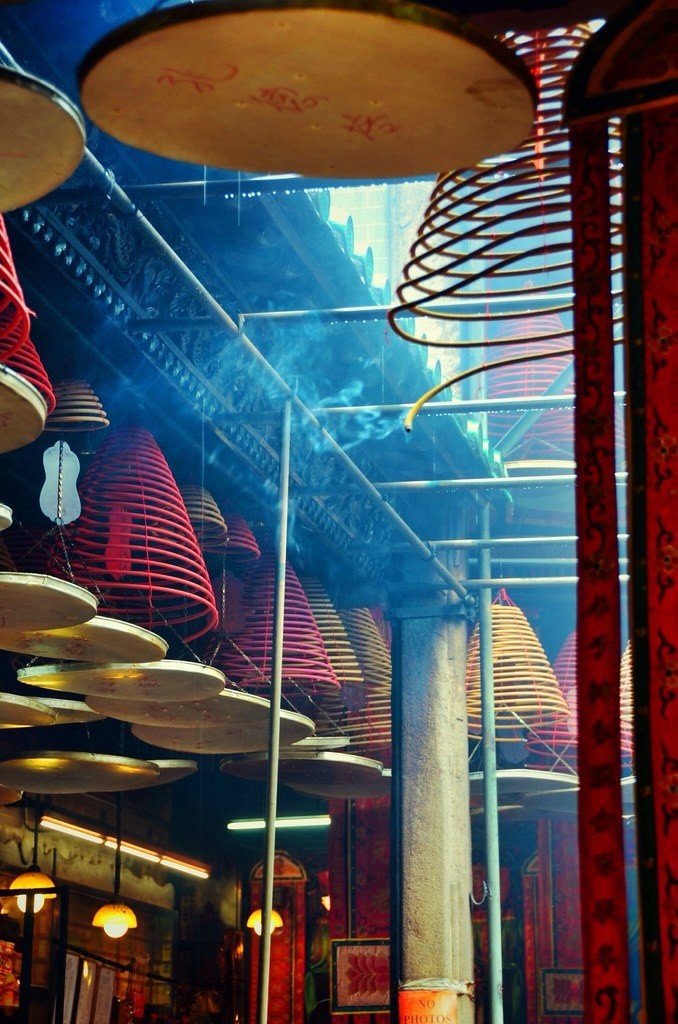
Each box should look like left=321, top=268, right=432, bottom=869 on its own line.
left=8, top=796, right=56, bottom=914
left=247, top=908, right=285, bottom=936
left=38, top=815, right=213, bottom=881
left=226, top=815, right=331, bottom=830
left=92, top=792, right=137, bottom=938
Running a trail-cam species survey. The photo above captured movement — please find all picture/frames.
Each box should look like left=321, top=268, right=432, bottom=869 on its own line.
left=330, top=937, right=391, bottom=1013
left=540, top=969, right=586, bottom=1015
